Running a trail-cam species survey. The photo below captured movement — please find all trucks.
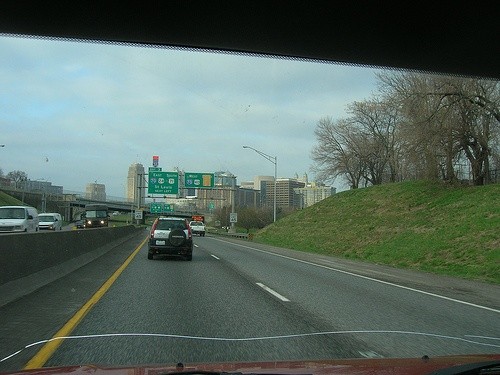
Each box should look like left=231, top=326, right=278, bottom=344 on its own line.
left=80, top=204, right=111, bottom=229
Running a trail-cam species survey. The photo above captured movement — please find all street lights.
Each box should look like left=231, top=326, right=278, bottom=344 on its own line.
left=243, top=145, right=277, bottom=223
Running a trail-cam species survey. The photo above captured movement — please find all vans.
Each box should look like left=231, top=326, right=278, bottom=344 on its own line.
left=37, top=212, right=62, bottom=231
left=0, top=206, right=40, bottom=233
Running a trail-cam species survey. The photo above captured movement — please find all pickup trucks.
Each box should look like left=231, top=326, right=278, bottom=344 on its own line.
left=189, top=221, right=206, bottom=237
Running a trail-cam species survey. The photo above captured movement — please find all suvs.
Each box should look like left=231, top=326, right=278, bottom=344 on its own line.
left=148, top=216, right=193, bottom=261
